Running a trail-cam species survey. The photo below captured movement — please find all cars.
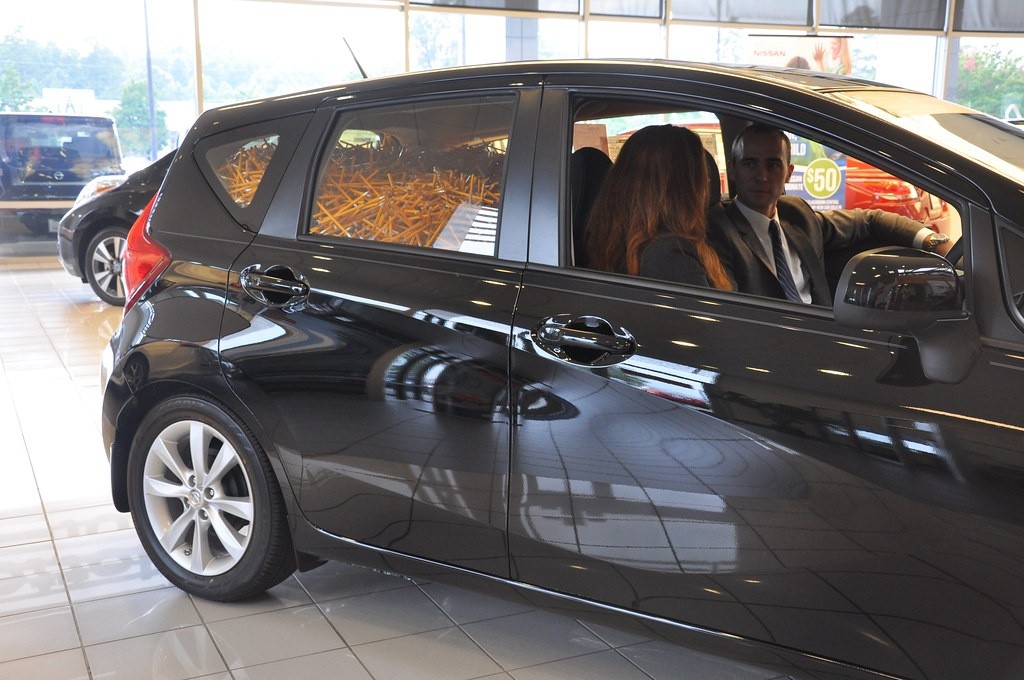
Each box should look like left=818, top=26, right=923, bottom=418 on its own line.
left=101, top=57, right=1023, bottom=680
left=55, top=130, right=185, bottom=309
left=2, top=113, right=124, bottom=211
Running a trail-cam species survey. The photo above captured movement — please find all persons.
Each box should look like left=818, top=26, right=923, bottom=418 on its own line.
left=582, top=124, right=734, bottom=292
left=706, top=124, right=955, bottom=309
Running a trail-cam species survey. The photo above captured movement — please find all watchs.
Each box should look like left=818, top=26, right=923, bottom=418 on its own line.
left=927, top=233, right=950, bottom=252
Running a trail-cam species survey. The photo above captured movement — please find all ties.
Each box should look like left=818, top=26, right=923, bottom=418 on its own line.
left=768, top=219, right=803, bottom=304
